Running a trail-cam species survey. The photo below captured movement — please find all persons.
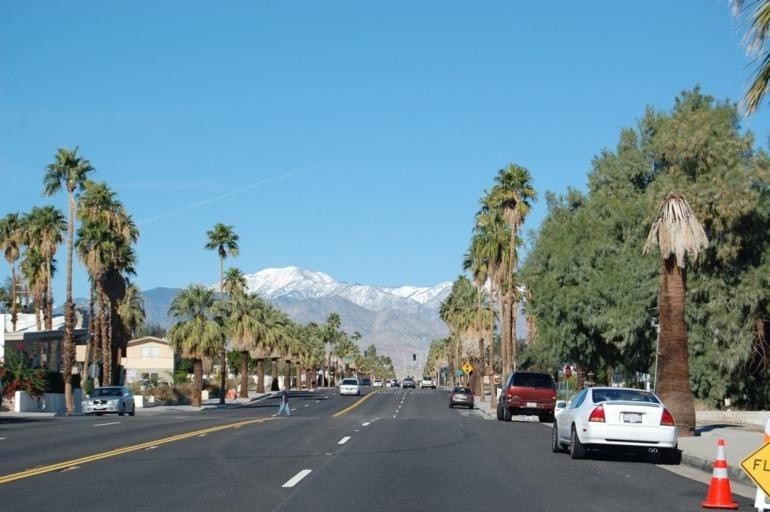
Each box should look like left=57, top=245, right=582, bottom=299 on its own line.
left=225, top=383, right=237, bottom=402
left=276, top=386, right=291, bottom=416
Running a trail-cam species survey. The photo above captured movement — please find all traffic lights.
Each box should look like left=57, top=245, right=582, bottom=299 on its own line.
left=413, top=354, right=416, bottom=361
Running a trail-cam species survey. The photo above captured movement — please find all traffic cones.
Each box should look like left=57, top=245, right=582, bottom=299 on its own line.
left=702, top=440, right=738, bottom=510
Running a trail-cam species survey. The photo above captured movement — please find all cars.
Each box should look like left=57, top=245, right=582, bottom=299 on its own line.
left=339, top=378, right=360, bottom=396
left=373, top=377, right=437, bottom=390
left=497, top=370, right=557, bottom=422
left=448, top=387, right=475, bottom=409
left=552, top=386, right=678, bottom=464
left=362, top=379, right=370, bottom=386
left=82, top=386, right=136, bottom=416
